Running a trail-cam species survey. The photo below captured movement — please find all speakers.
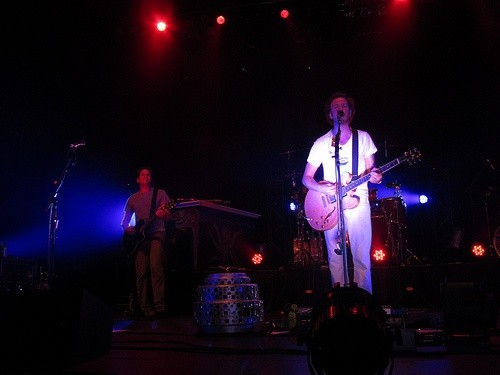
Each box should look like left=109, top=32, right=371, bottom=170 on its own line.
left=33, top=290, right=115, bottom=356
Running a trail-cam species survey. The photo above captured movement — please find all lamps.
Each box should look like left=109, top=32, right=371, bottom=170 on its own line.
left=296, top=286, right=403, bottom=375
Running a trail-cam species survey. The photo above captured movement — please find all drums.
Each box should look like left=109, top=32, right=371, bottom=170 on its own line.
left=293, top=237, right=324, bottom=265
left=379, top=197, right=404, bottom=225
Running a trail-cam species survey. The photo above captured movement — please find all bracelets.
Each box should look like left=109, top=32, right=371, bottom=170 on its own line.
left=377, top=177, right=383, bottom=184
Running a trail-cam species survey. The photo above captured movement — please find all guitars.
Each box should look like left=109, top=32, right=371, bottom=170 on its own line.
left=304, top=149, right=422, bottom=231
left=122, top=199, right=178, bottom=258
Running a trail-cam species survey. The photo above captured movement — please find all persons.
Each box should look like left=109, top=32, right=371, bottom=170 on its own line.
left=302, top=94, right=382, bottom=295
left=122, top=170, right=170, bottom=324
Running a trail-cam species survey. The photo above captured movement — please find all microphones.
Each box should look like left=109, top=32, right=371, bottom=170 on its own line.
left=336, top=110, right=344, bottom=118
